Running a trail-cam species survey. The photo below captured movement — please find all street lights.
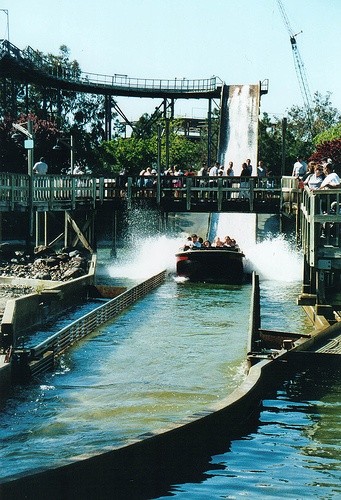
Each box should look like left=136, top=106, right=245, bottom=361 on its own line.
left=12, top=118, right=34, bottom=175
left=156, top=122, right=166, bottom=175
left=53, top=135, right=73, bottom=175
left=0, top=9, right=9, bottom=43
left=282, top=118, right=287, bottom=175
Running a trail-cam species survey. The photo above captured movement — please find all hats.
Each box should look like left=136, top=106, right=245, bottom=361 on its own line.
left=296, top=155, right=302, bottom=159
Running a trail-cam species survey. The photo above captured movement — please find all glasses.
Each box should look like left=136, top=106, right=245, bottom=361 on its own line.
left=317, top=169, right=323, bottom=171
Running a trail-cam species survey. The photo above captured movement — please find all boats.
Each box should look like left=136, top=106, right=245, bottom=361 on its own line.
left=1, top=212, right=98, bottom=347
left=176, top=246, right=245, bottom=283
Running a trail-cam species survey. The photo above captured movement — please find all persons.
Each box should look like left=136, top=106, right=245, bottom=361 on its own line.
left=256, top=161, right=269, bottom=203
left=226, top=162, right=235, bottom=199
left=302, top=162, right=317, bottom=184
left=198, top=162, right=208, bottom=202
left=238, top=163, right=251, bottom=201
left=300, top=164, right=325, bottom=214
left=320, top=157, right=331, bottom=175
left=60, top=159, right=223, bottom=198
left=317, top=163, right=341, bottom=216
left=293, top=155, right=307, bottom=178
left=181, top=233, right=240, bottom=252
left=245, top=159, right=253, bottom=176
left=33, top=157, right=48, bottom=175
left=208, top=162, right=221, bottom=202
left=218, top=165, right=225, bottom=176
